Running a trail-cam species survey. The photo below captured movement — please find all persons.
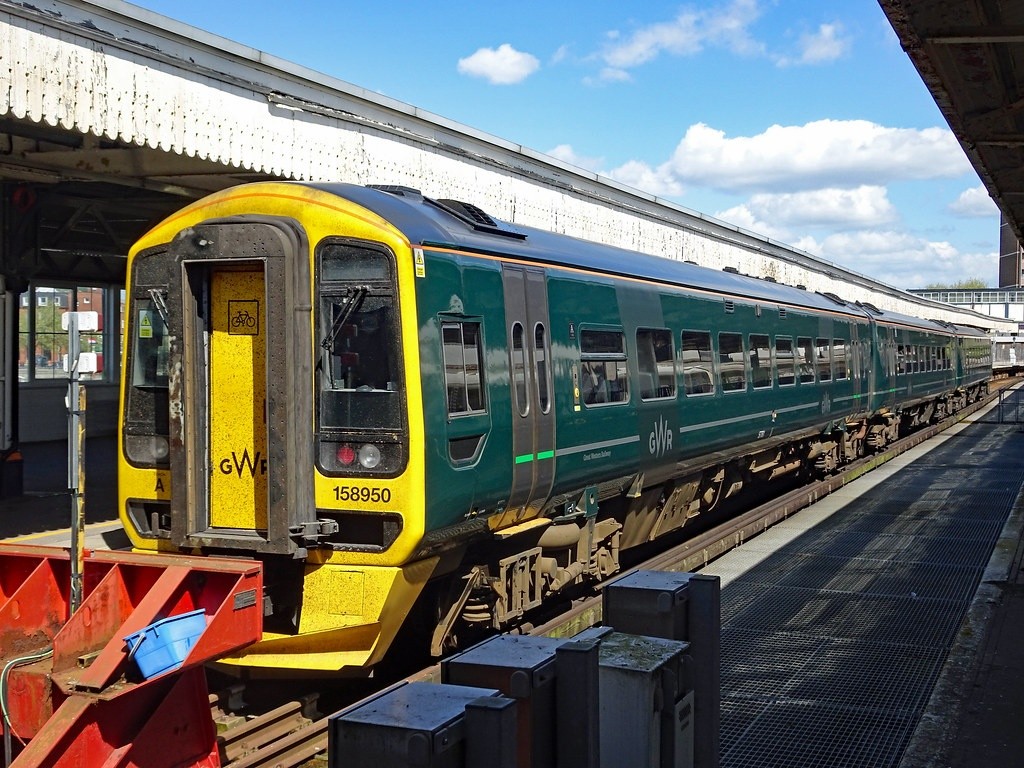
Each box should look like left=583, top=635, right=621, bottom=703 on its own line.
left=582, top=364, right=608, bottom=402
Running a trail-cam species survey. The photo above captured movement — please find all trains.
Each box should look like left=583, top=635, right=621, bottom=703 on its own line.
left=117, top=182, right=997, bottom=683
